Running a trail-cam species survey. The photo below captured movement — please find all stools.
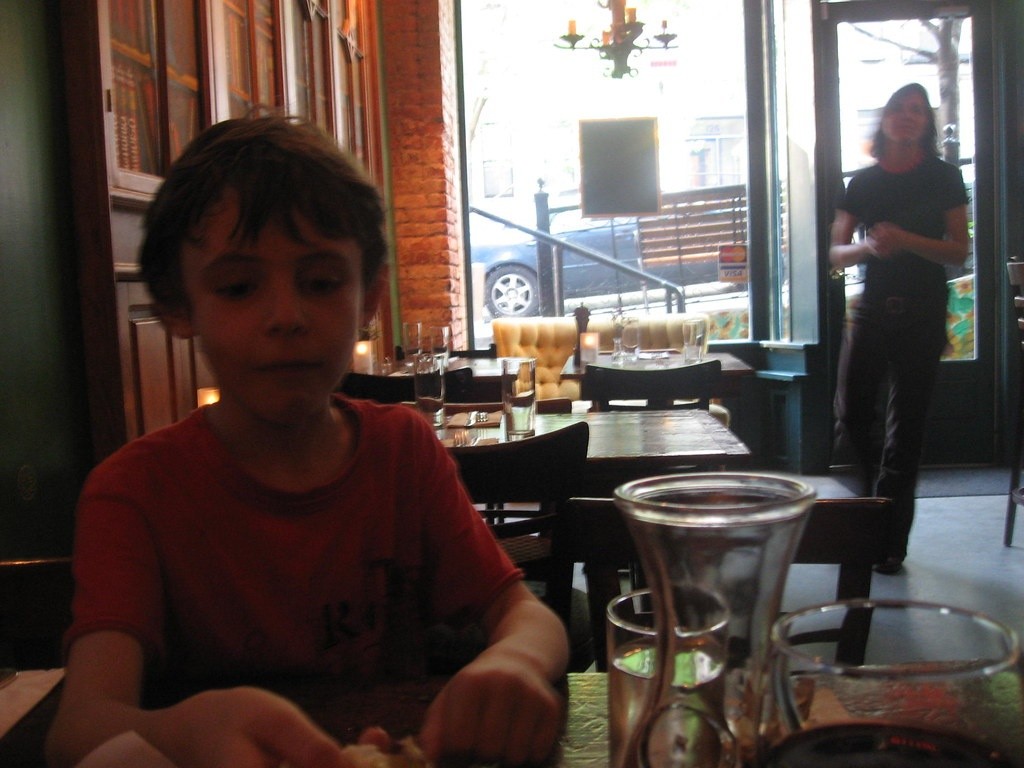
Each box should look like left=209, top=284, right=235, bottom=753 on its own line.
left=1002, top=255, right=1024, bottom=548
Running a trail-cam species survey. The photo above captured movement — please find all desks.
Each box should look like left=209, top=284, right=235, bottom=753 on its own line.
left=0, top=659, right=1024, bottom=768
left=416, top=405, right=748, bottom=668
left=380, top=348, right=754, bottom=407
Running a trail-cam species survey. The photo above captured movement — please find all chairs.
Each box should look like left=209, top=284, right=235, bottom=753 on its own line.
left=340, top=360, right=895, bottom=669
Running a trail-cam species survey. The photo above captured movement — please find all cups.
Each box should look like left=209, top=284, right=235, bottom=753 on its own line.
left=756, top=599, right=1024, bottom=767
left=402, top=322, right=422, bottom=367
left=429, top=326, right=449, bottom=357
left=682, top=322, right=703, bottom=364
left=607, top=579, right=732, bottom=768
left=620, top=317, right=640, bottom=358
left=500, top=356, right=538, bottom=443
left=413, top=353, right=446, bottom=428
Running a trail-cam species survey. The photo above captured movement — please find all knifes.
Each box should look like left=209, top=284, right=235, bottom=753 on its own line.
left=466, top=410, right=479, bottom=427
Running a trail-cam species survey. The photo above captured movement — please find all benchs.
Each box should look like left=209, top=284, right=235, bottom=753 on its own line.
left=491, top=315, right=712, bottom=408
left=635, top=180, right=784, bottom=311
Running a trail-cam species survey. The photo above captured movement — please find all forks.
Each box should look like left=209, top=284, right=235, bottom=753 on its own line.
left=476, top=411, right=488, bottom=421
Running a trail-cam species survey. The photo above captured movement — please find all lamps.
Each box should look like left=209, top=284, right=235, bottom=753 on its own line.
left=554, top=0, right=678, bottom=79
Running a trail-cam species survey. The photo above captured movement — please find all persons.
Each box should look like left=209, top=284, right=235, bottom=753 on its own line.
left=48, top=102, right=569, bottom=768
left=828, top=82, right=968, bottom=576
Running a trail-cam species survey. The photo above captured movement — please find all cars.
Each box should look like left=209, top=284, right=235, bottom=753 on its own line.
left=469, top=187, right=748, bottom=319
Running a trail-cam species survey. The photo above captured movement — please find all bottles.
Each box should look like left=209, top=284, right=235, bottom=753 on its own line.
left=609, top=470, right=822, bottom=768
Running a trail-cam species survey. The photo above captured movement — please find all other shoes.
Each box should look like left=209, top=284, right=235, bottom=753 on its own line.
left=873, top=557, right=902, bottom=575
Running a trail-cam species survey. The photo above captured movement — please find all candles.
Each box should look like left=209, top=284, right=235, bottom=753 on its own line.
left=351, top=341, right=373, bottom=373
left=580, top=332, right=600, bottom=364
left=197, top=387, right=221, bottom=407
